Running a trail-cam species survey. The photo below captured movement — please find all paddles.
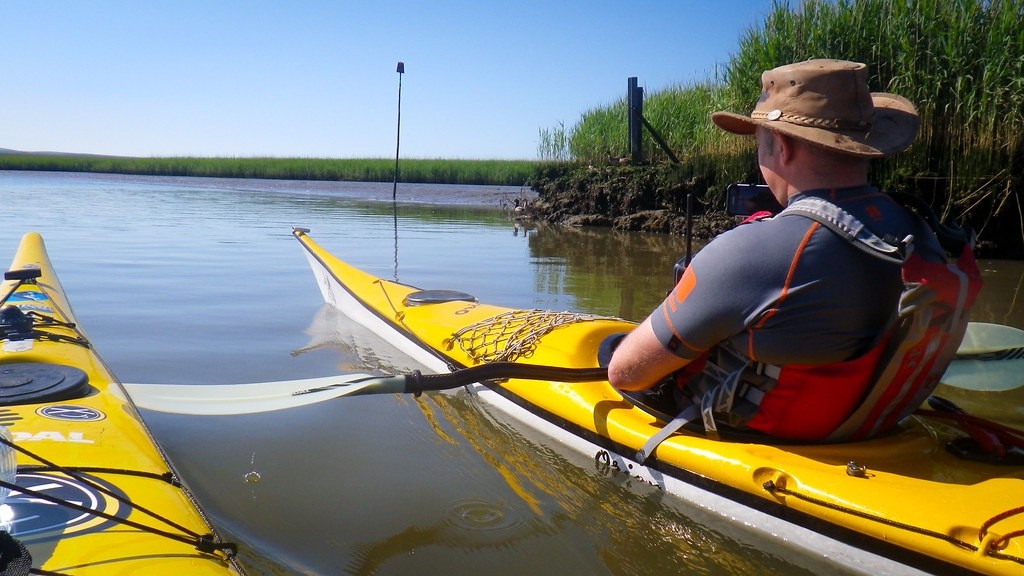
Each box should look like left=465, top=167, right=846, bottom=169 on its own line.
left=112, top=315, right=1024, bottom=415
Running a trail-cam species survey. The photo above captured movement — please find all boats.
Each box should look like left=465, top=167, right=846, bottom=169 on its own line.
left=0, top=232, right=249, bottom=576
left=290, top=223, right=1024, bottom=576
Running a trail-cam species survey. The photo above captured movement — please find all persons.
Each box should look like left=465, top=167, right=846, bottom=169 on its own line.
left=608, top=59, right=982, bottom=444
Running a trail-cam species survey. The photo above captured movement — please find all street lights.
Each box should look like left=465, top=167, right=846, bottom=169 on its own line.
left=393, top=62, right=406, bottom=202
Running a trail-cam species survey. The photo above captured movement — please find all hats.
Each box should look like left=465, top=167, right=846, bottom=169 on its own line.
left=712, top=59, right=920, bottom=157
